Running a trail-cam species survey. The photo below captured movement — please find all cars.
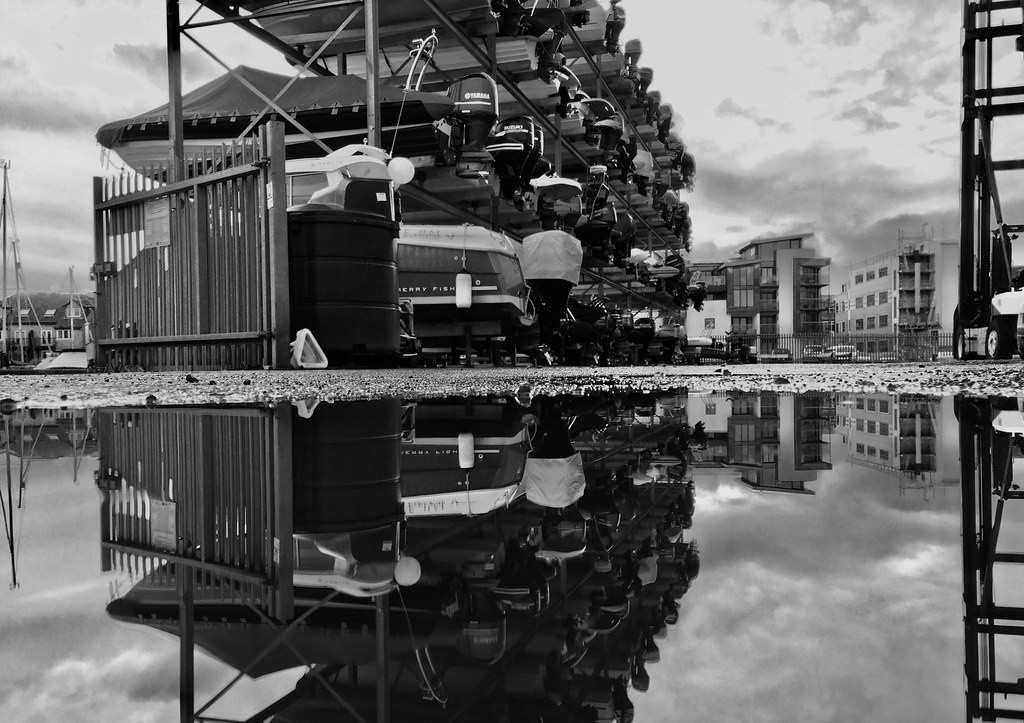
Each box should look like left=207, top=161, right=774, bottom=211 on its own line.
left=771, top=345, right=898, bottom=363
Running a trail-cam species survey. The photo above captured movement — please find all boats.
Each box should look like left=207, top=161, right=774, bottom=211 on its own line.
left=105, top=392, right=701, bottom=723
left=96, top=65, right=499, bottom=173
left=391, top=224, right=688, bottom=367
left=245, top=1, right=708, bottom=313
